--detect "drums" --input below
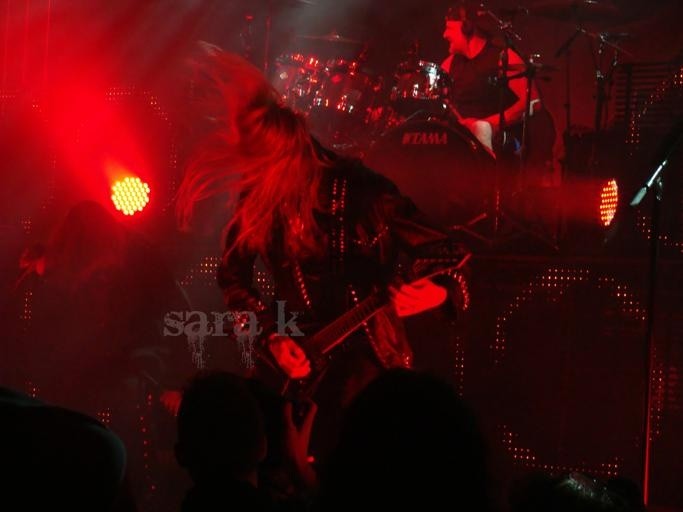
[304,59,384,152]
[391,60,454,116]
[369,123,478,227]
[272,51,324,109]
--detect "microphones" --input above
[627,152,671,207]
[553,31,579,58]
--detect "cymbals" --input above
[304,35,360,48]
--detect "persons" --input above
[170,369,304,510]
[424,3,543,178]
[0,385,128,510]
[293,365,503,511]
[174,47,485,471]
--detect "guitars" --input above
[159,254,471,488]
